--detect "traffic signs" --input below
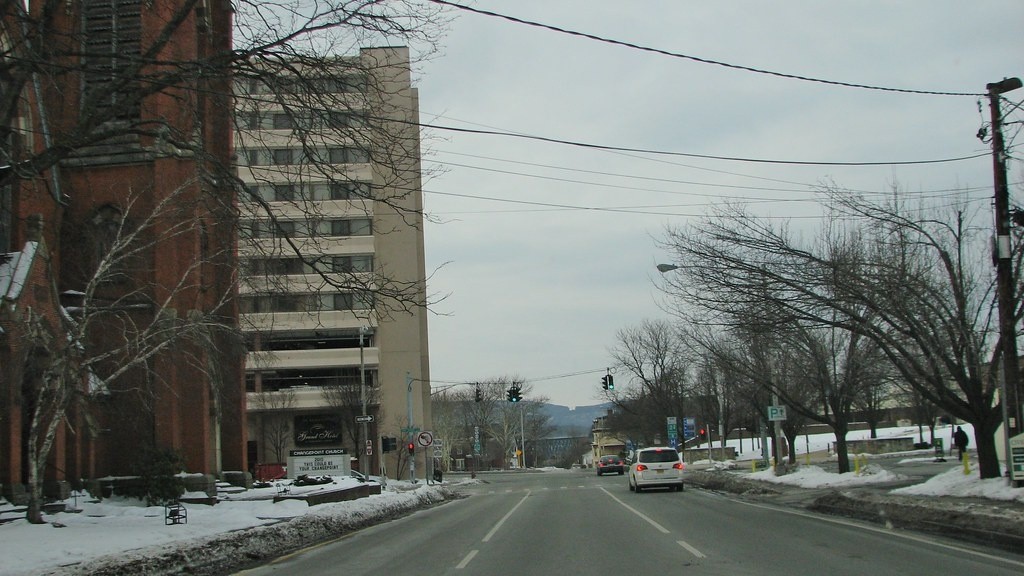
[356,415,374,423]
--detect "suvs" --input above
[629,446,685,491]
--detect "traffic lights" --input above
[609,376,614,389]
[507,388,523,403]
[382,437,397,453]
[701,428,706,441]
[476,388,481,402]
[602,377,608,390]
[409,443,415,456]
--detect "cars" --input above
[351,470,386,489]
[596,455,625,476]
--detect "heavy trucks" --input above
[255,458,357,481]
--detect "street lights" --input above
[520,401,525,467]
[658,263,790,479]
[359,324,371,482]
[600,406,604,454]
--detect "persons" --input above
[955,426,968,460]
